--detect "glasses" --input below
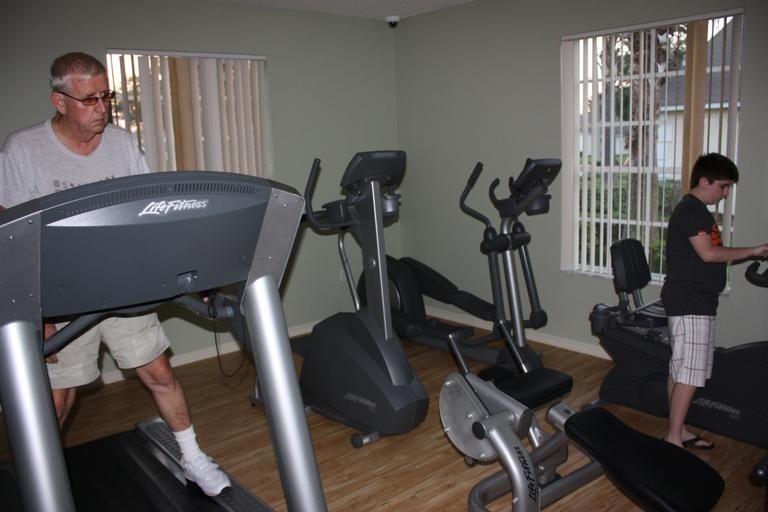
[58,90,115,107]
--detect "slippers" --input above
[682,434,715,450]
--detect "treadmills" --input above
[0,167,325,512]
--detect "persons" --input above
[658,152,768,449]
[0,51,233,499]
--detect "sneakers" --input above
[180,451,232,498]
